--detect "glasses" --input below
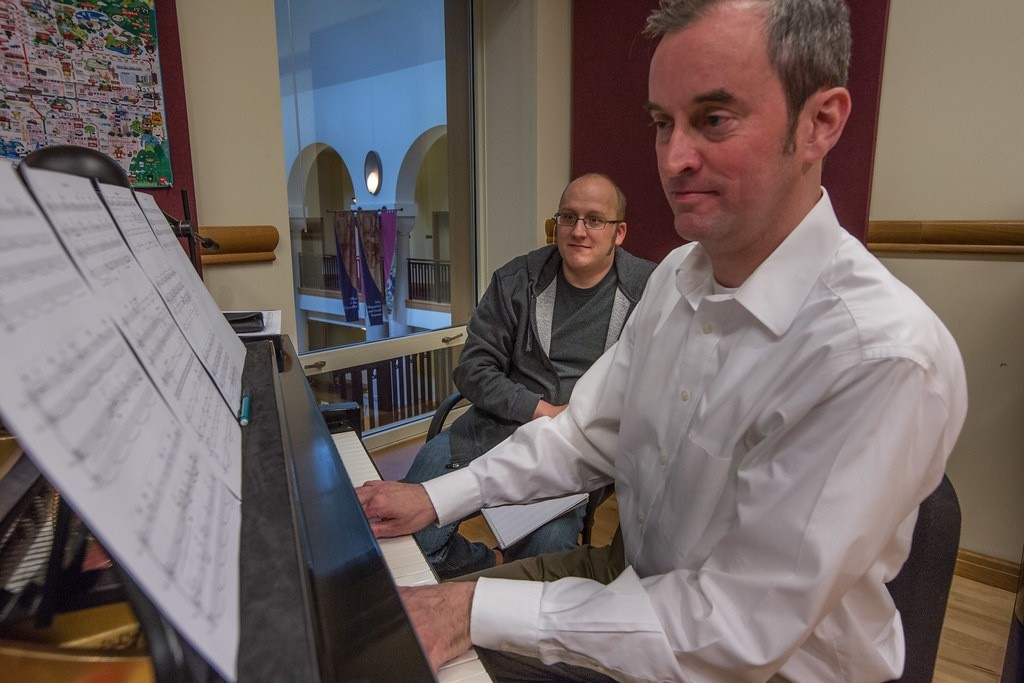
[553,212,623,230]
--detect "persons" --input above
[393,173,660,585]
[354,0,970,683]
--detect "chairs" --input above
[863,469,962,682]
[425,395,619,546]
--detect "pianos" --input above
[1,144,499,682]
